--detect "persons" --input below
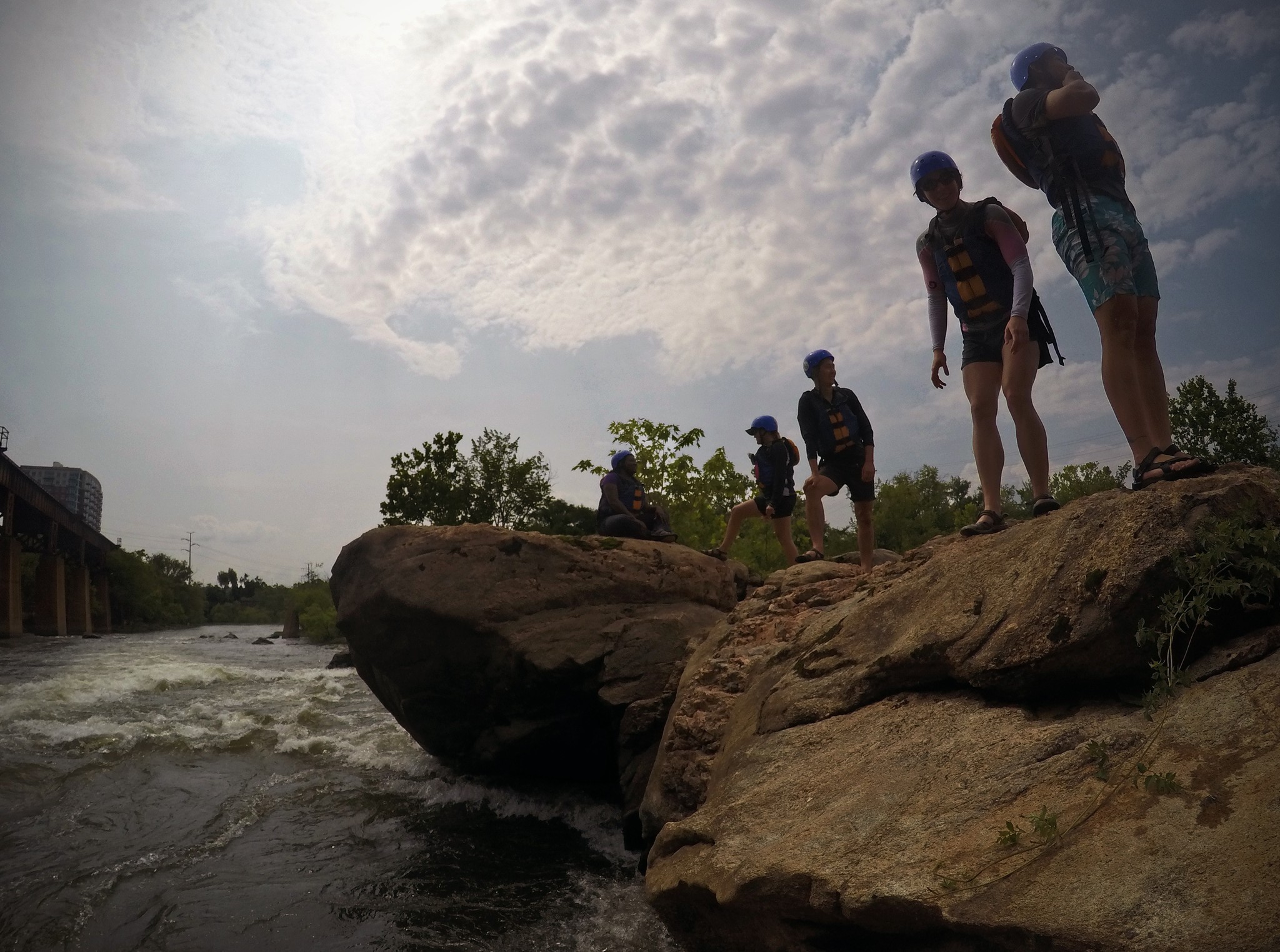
[597,450,679,543]
[700,415,799,569]
[795,350,876,573]
[1001,42,1209,493]
[910,151,1066,538]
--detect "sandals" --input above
[795,548,825,563]
[1034,495,1061,517]
[650,528,677,543]
[960,510,1008,536]
[1132,447,1202,491]
[1164,445,1216,475]
[700,548,727,561]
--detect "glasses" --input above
[919,172,956,191]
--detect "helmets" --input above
[911,151,963,202]
[611,450,635,470]
[803,350,834,378]
[1010,42,1067,92]
[745,415,778,436]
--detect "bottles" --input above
[754,461,759,478]
[633,482,642,510]
